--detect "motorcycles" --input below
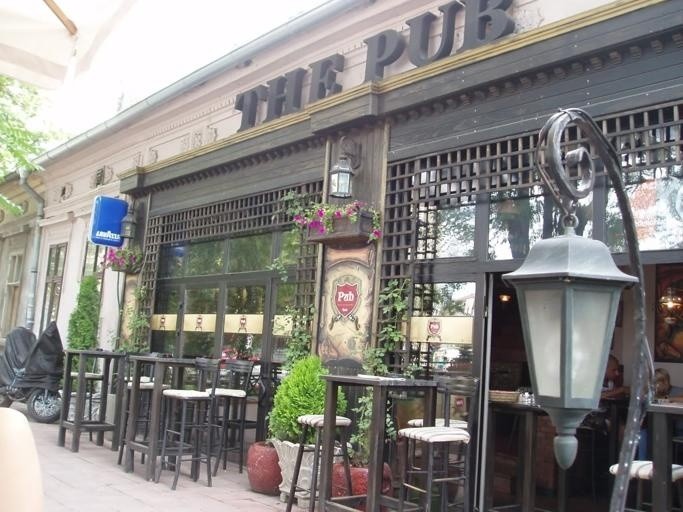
[1,320,63,424]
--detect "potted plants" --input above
[269,352,397,512]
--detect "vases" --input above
[248,439,282,494]
[111,264,131,272]
[305,212,377,243]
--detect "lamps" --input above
[120,204,139,239]
[329,133,363,199]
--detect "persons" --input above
[591,353,631,463]
[654,368,682,403]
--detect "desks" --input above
[318,369,436,511]
[59,349,124,452]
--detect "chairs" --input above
[71,349,281,491]
[400,373,681,511]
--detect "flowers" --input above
[266,190,382,282]
[100,244,142,275]
